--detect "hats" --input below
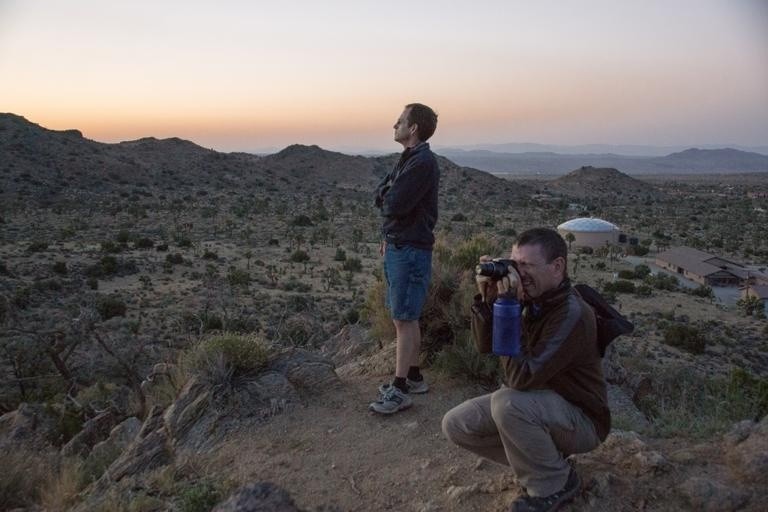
[573,284,635,359]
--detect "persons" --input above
[438,225,613,510]
[367,102,439,417]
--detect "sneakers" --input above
[507,467,582,512]
[369,384,414,415]
[378,376,430,396]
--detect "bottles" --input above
[492,298,520,356]
